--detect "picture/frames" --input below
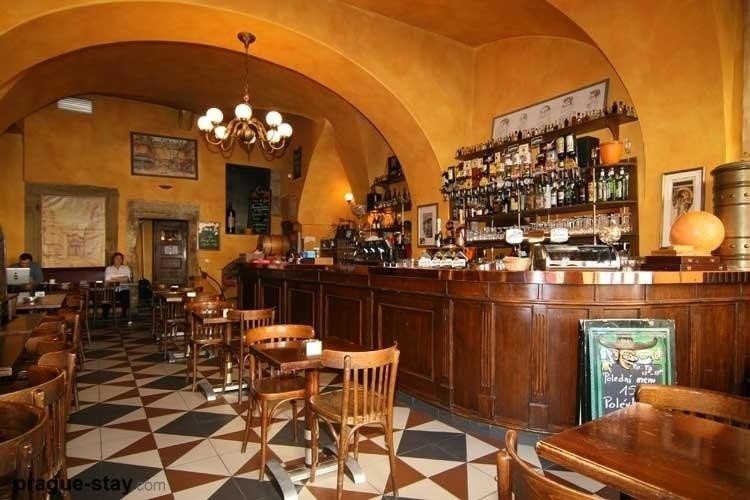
[659,166,706,249]
[130,130,199,179]
[491,78,610,144]
[197,220,220,250]
[416,203,438,248]
[576,319,677,427]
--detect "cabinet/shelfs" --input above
[442,113,640,270]
[364,174,412,236]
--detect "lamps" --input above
[345,193,353,205]
[196,33,294,155]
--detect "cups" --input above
[621,257,641,272]
[466,212,631,244]
[160,285,165,289]
[395,258,418,268]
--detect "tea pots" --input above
[330,239,337,265]
[320,239,329,248]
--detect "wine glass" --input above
[383,232,402,267]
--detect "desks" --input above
[535,401,750,500]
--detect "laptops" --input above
[5,268,31,284]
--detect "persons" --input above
[422,214,432,237]
[105,253,132,322]
[7,254,44,294]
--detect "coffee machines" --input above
[351,240,405,266]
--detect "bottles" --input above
[455,209,466,248]
[374,175,385,184]
[434,218,443,247]
[225,200,236,234]
[372,212,399,229]
[440,99,637,220]
[374,186,408,209]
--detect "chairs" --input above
[494,430,607,500]
[0,278,130,500]
[147,274,400,500]
[634,383,749,428]
